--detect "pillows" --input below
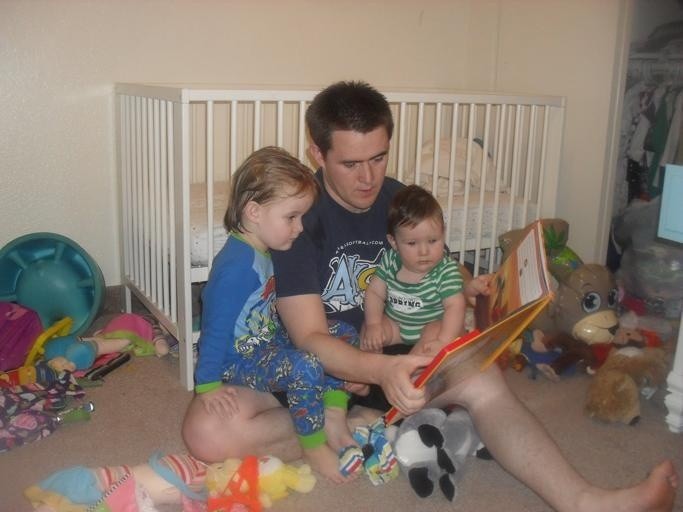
[412,134,478,184]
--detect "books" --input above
[382,217,554,425]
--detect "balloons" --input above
[497,217,617,346]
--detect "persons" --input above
[356,184,469,361]
[178,79,677,512]
[191,145,360,485]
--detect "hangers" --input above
[625,53,681,100]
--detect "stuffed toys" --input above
[23,451,207,512]
[383,405,493,503]
[202,455,313,512]
[504,319,668,432]
[43,311,178,373]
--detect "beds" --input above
[111,76,568,391]
